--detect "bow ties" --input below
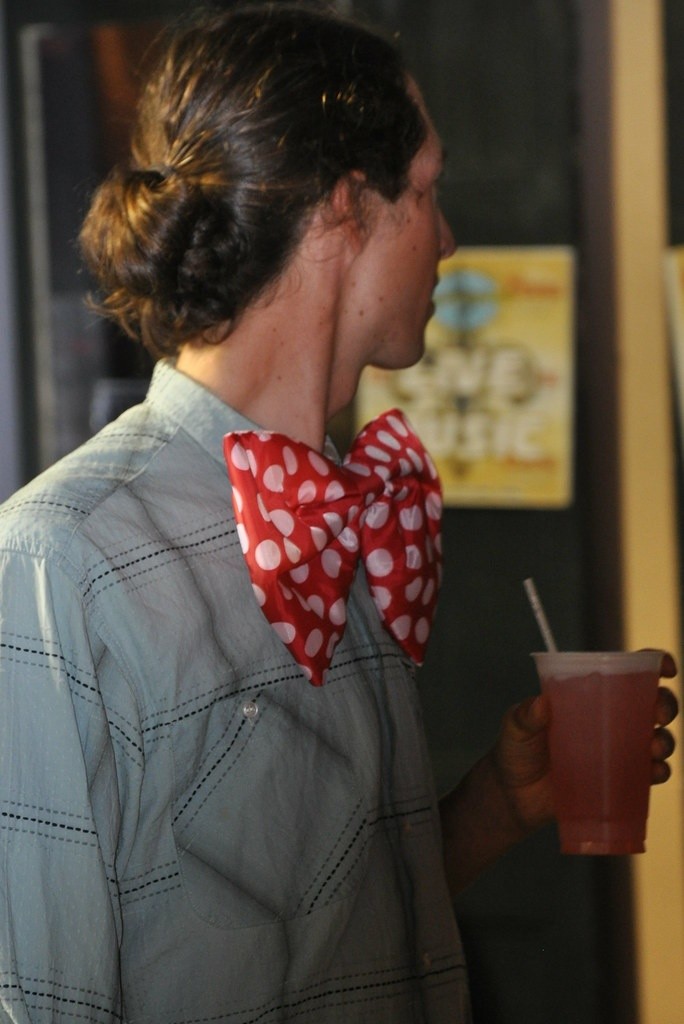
[225,407,448,690]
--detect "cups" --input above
[528,649,665,856]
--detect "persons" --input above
[0,1,679,1023]
